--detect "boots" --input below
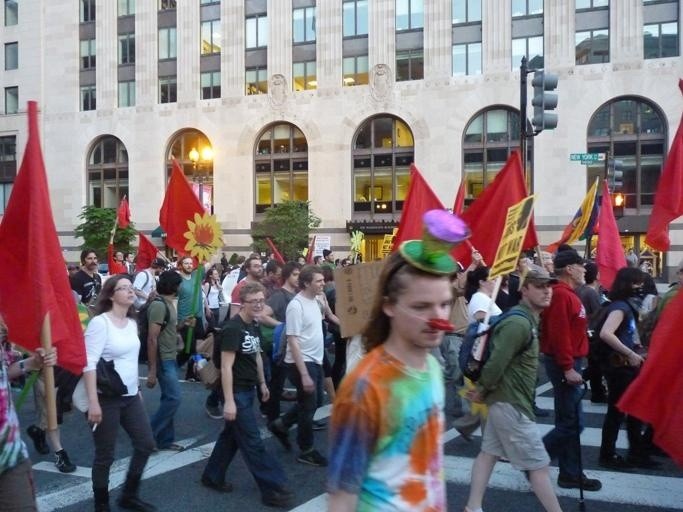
[117,472,156,512]
[93,482,113,512]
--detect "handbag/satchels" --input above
[259,313,276,355]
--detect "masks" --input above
[632,286,645,296]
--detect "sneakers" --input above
[590,392,608,403]
[598,452,638,472]
[556,471,601,491]
[452,419,475,441]
[626,450,664,471]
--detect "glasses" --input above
[244,299,265,305]
[115,287,134,290]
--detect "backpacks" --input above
[189,320,244,389]
[587,302,615,365]
[136,299,169,363]
[458,311,533,382]
[272,298,303,364]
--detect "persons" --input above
[0,228,682,512]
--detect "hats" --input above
[153,258,170,269]
[553,250,589,266]
[323,249,332,257]
[525,270,559,287]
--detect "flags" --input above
[543,176,600,254]
[158,159,212,273]
[595,178,628,294]
[389,163,474,275]
[0,100,87,376]
[456,148,539,269]
[117,198,131,232]
[450,180,464,217]
[641,110,683,254]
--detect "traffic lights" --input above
[535,69,562,130]
[610,158,625,188]
[614,192,625,216]
